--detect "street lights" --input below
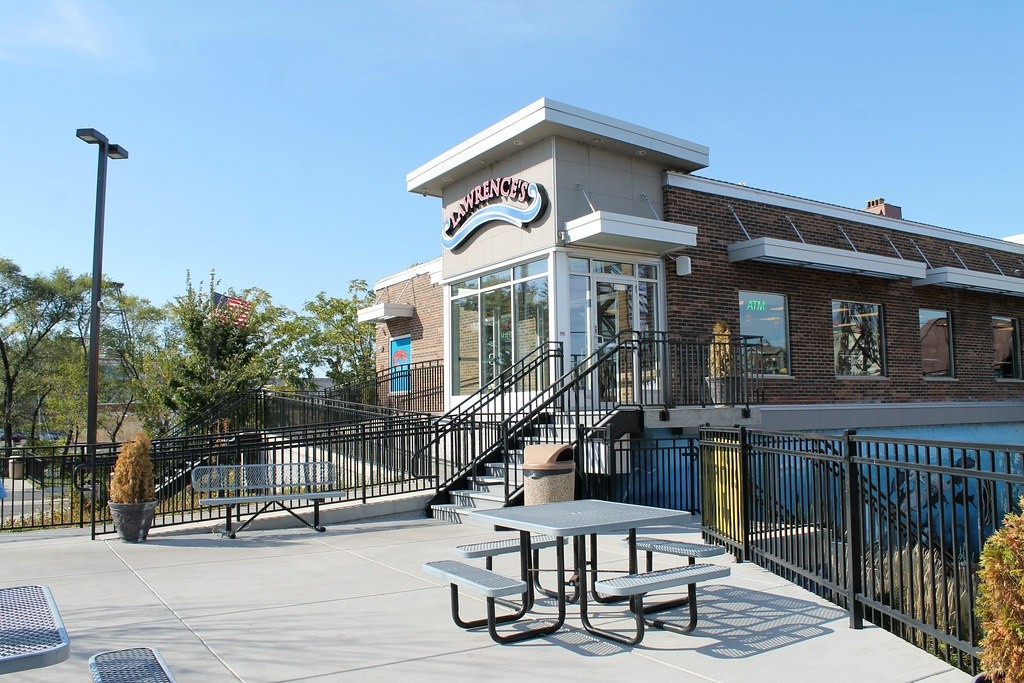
[75,126,130,485]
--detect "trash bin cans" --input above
[9,455,23,479]
[522,444,576,505]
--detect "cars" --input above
[0,428,67,445]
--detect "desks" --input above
[0,585,70,675]
[469,499,692,628]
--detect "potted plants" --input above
[706,321,743,407]
[108,431,158,544]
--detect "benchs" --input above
[88,647,176,683]
[192,462,346,538]
[425,535,732,647]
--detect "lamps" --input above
[423,189,429,197]
[558,230,565,241]
